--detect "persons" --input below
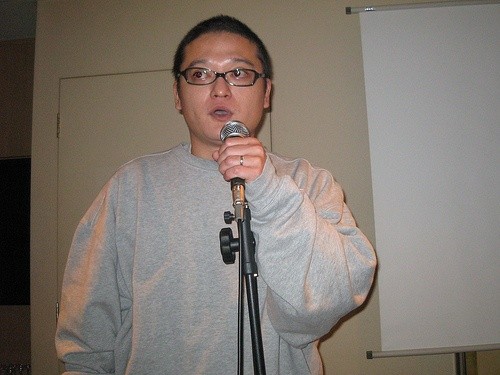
[53,13,378,374]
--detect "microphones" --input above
[220,119,251,221]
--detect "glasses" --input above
[177,67,267,87]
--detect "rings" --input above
[240,156,244,166]
